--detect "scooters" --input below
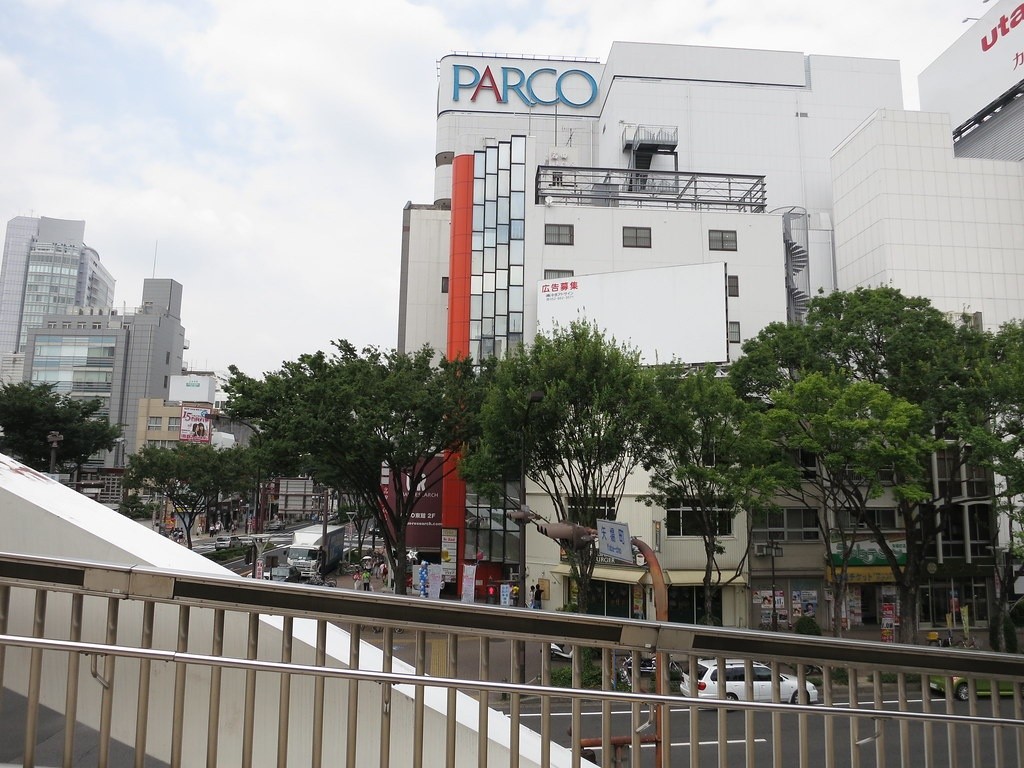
[551,642,573,661]
[622,652,683,680]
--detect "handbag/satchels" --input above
[509,589,519,598]
[356,579,360,587]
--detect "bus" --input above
[361,555,372,571]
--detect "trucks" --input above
[283,524,345,579]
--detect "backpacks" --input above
[383,565,388,574]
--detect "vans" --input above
[214,536,243,551]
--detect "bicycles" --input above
[927,630,954,647]
[303,573,338,587]
[954,634,979,650]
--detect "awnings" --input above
[662,571,750,588]
[548,562,647,592]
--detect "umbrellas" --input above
[362,556,372,559]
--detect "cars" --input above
[680,658,818,710]
[267,520,285,531]
[926,674,1024,700]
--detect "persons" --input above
[529,584,544,609]
[192,423,208,436]
[353,552,387,591]
[197,521,220,537]
[753,591,816,618]
[169,527,183,542]
[228,520,238,536]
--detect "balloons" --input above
[418,561,428,598]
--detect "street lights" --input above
[204,413,262,579]
[763,538,784,635]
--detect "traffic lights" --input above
[504,511,529,522]
[486,583,497,605]
[536,521,587,543]
